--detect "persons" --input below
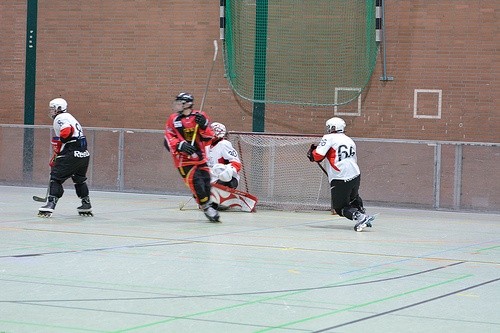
[163,92,222,223]
[36,97,94,218]
[203,120,242,190]
[306,117,375,232]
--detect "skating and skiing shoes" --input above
[38,196,58,218]
[200,203,220,222]
[77,197,93,216]
[353,212,375,232]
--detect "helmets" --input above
[211,122,226,138]
[326,117,346,133]
[176,93,194,102]
[49,98,67,112]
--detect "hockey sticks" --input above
[33,158,57,202]
[179,178,218,211]
[317,161,371,226]
[187,40,218,159]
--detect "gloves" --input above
[195,114,207,130]
[177,141,196,155]
[307,144,316,162]
[52,140,57,152]
